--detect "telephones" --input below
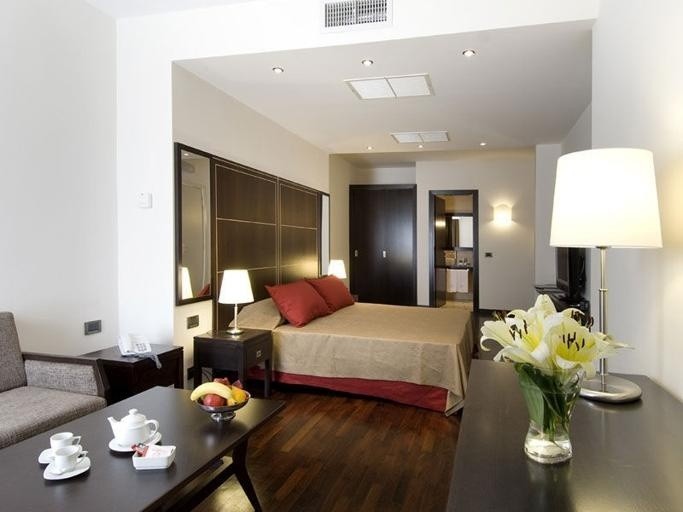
[118,332,152,357]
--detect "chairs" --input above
[0,310,107,448]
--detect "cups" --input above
[49,430,81,450]
[53,447,86,468]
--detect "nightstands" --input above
[351,293,359,303]
[192,324,275,400]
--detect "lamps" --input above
[181,266,194,302]
[550,146,661,408]
[488,201,516,230]
[217,268,254,334]
[327,258,347,281]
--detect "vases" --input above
[517,362,580,461]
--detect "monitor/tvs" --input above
[555,247,585,299]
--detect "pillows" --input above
[308,273,354,311]
[263,277,333,328]
[197,284,210,297]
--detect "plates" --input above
[43,458,90,481]
[106,433,163,454]
[36,445,83,464]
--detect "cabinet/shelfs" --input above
[348,184,417,307]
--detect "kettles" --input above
[106,407,159,445]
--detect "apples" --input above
[230,380,242,391]
[203,394,225,406]
[214,378,229,386]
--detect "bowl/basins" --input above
[131,445,177,470]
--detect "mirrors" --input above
[173,140,214,307]
[447,214,473,250]
[320,191,330,278]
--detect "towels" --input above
[442,269,469,295]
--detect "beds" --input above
[226,286,473,416]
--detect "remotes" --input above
[543,289,561,292]
[535,285,557,289]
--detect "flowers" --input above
[474,292,631,370]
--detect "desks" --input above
[445,357,681,512]
[80,341,183,404]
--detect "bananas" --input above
[231,386,245,405]
[190,382,236,406]
